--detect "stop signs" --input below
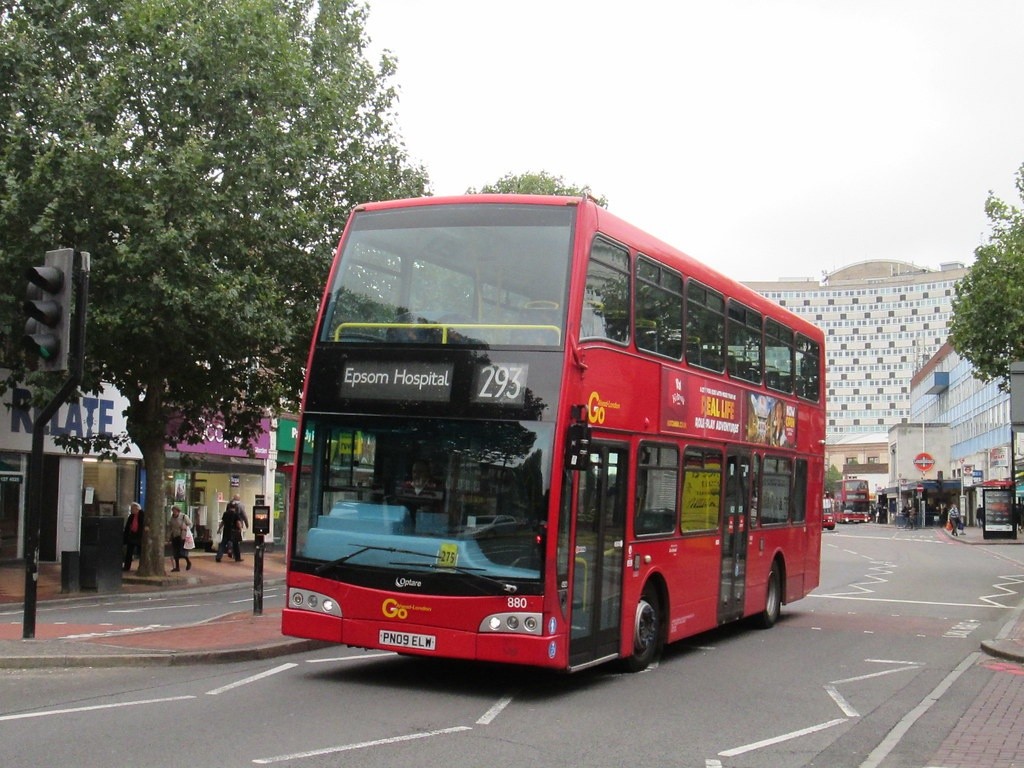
[916,483,924,493]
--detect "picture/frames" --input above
[96,501,116,516]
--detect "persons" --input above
[167,503,197,573]
[122,499,144,573]
[214,493,248,562]
[899,506,920,529]
[1016,498,1024,533]
[976,504,983,527]
[771,398,788,448]
[399,458,442,491]
[933,503,962,537]
[870,503,888,524]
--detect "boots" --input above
[184,557,192,570]
[171,562,180,571]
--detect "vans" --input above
[822,495,836,531]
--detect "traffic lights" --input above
[24,248,75,374]
[22,262,45,369]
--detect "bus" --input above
[279,192,827,675]
[832,478,871,524]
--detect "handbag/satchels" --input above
[946,522,952,531]
[231,530,242,542]
[183,525,195,549]
[958,522,963,530]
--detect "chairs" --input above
[468,308,522,346]
[382,309,478,345]
[514,299,806,399]
[643,507,675,530]
[726,475,748,499]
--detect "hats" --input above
[171,504,182,511]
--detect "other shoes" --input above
[122,566,131,571]
[217,559,221,562]
[235,558,243,561]
